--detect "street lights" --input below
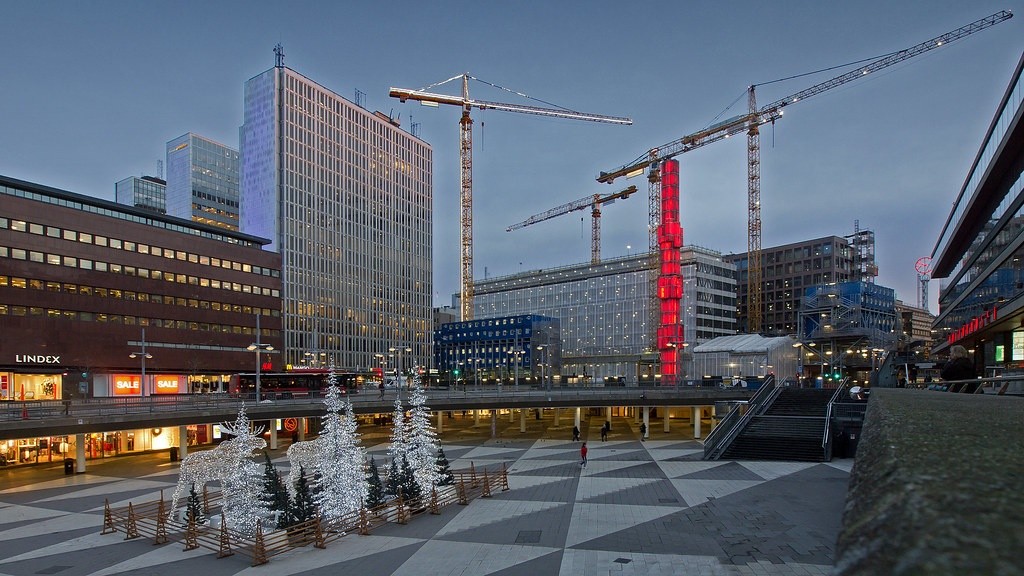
[129,352,153,400]
[508,349,526,386]
[537,344,551,391]
[247,343,274,404]
[389,346,412,400]
[375,354,385,385]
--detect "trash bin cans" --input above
[281,392,292,400]
[292,431,299,443]
[170,447,177,462]
[65,458,74,475]
[536,412,539,419]
[618,377,626,386]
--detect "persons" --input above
[292,429,300,443]
[940,345,984,394]
[601,426,608,442]
[60,394,73,415]
[910,367,918,383]
[579,443,587,468]
[377,382,385,400]
[572,426,580,441]
[639,423,646,441]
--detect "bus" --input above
[228,369,347,399]
[288,370,359,394]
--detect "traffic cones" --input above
[22,404,29,419]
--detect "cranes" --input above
[596,106,785,350]
[681,9,1014,331]
[389,71,633,319]
[508,185,639,264]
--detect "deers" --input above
[168,417,267,528]
